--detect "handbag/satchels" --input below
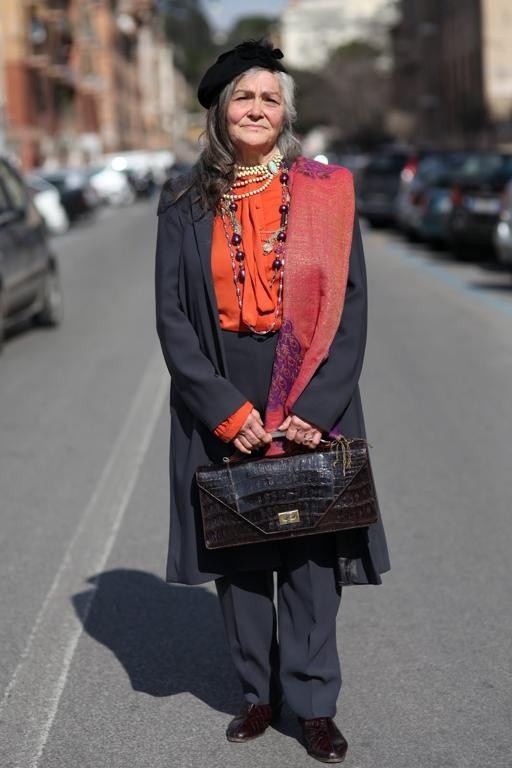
[189,429,380,552]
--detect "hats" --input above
[193,40,287,114]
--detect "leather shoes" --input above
[291,707,352,763]
[223,688,280,749]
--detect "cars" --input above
[311,139,512,268]
[0,146,178,337]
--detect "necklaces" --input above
[212,148,291,340]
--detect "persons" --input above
[153,34,393,764]
